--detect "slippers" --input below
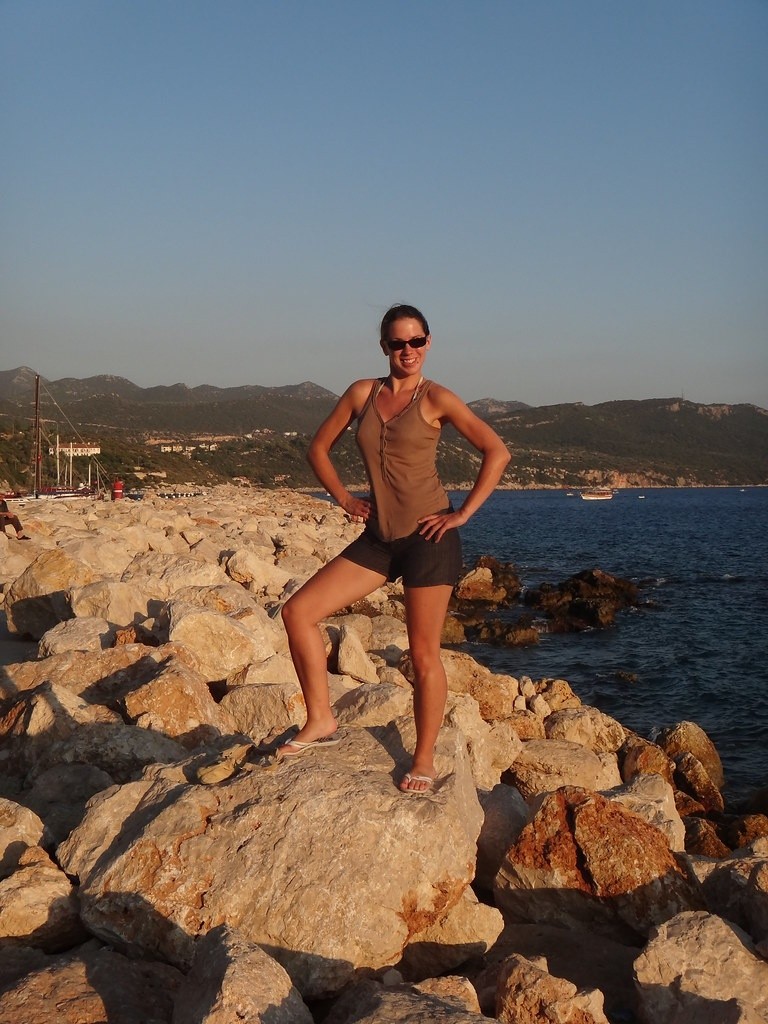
[399,772,435,794]
[279,733,341,756]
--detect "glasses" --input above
[384,334,427,351]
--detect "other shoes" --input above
[18,535,31,540]
[6,535,12,539]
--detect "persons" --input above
[273,304,513,793]
[0,489,31,540]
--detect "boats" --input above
[580,489,613,500]
